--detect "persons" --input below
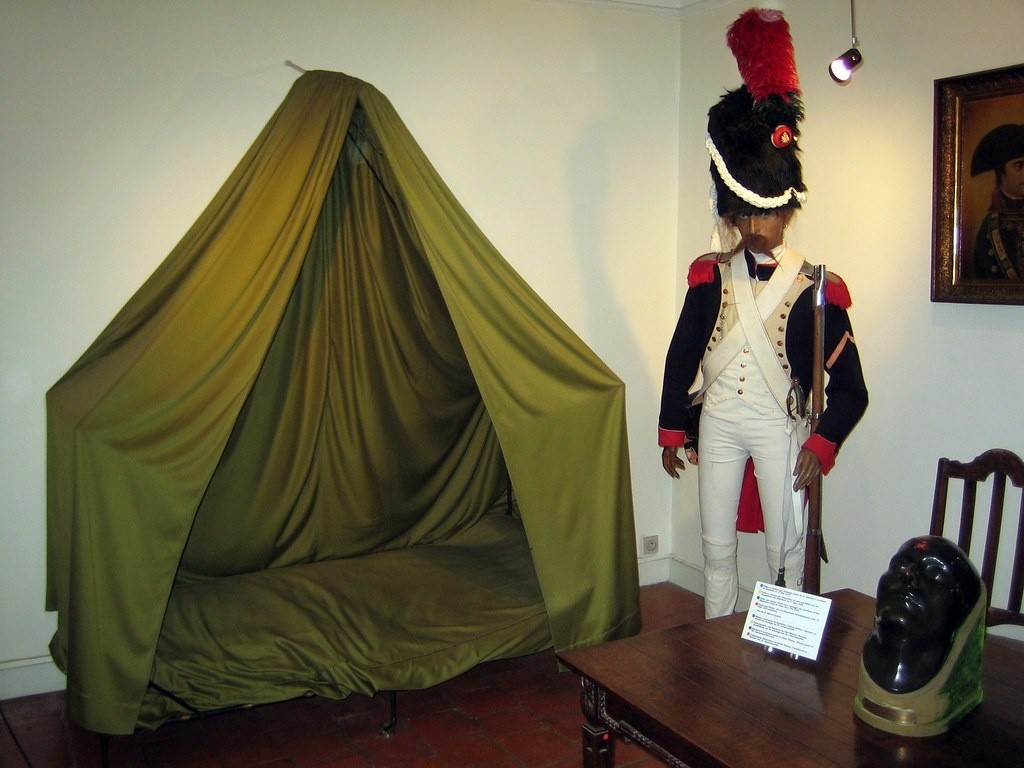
[656,7,869,620]
[855,534,988,738]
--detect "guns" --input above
[800,262,829,600]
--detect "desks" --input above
[556,589,1024,768]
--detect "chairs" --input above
[930,449,1024,629]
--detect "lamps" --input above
[828,0,862,82]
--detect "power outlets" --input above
[643,536,659,555]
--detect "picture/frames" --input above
[931,63,1024,306]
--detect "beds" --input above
[46,384,642,739]
